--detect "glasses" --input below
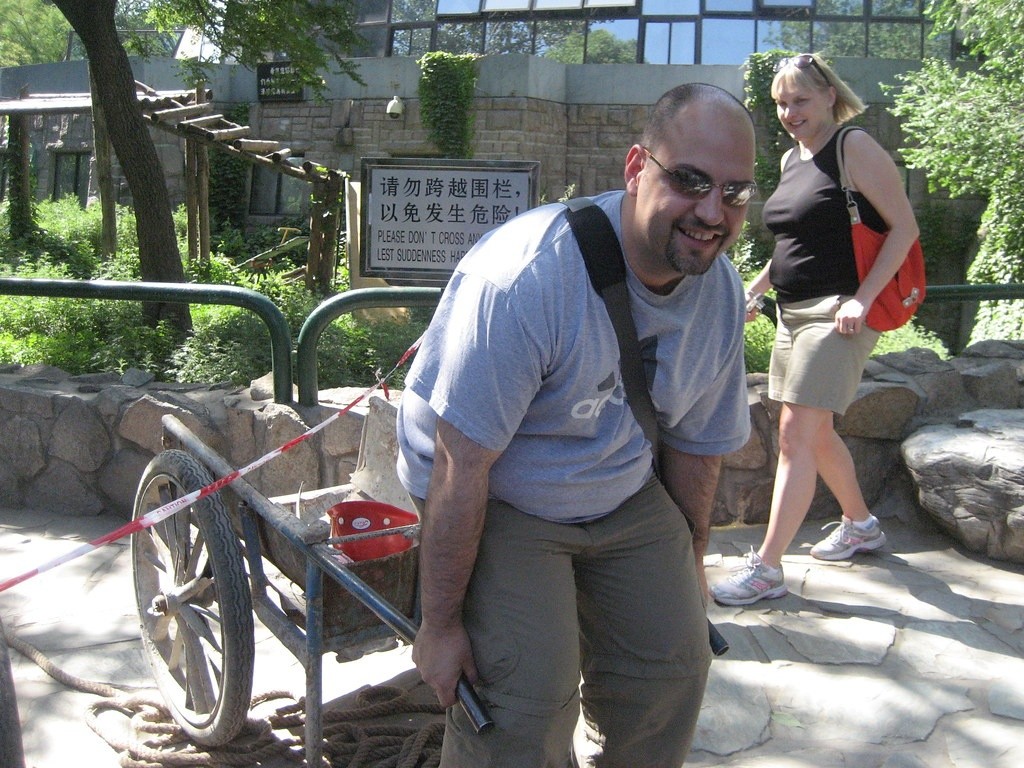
[772,55,831,88]
[641,146,758,206]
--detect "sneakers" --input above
[709,544,787,605]
[810,514,885,561]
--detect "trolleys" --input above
[128,412,731,768]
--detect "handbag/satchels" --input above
[836,125,926,331]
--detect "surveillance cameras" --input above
[386,100,402,119]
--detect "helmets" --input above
[327,501,420,561]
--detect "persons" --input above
[395,82,756,768]
[709,54,919,606]
